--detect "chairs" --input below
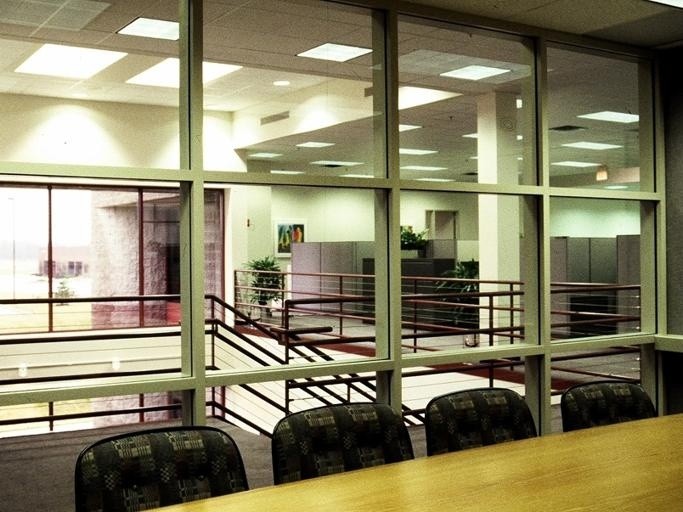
[75,425,249,511]
[560,382,657,433]
[271,401,415,486]
[423,387,538,457]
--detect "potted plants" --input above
[435,258,480,346]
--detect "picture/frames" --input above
[275,220,309,258]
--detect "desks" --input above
[140,411,683,512]
